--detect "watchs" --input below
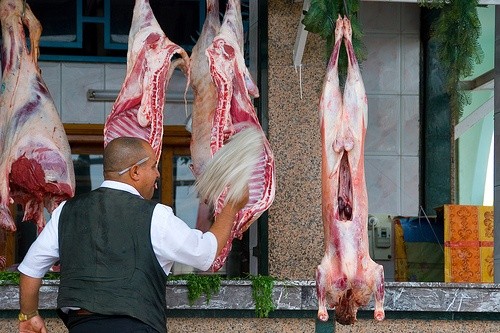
[19,310,38,321]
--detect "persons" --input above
[17,137,249,333]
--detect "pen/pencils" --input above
[119,156,150,176]
